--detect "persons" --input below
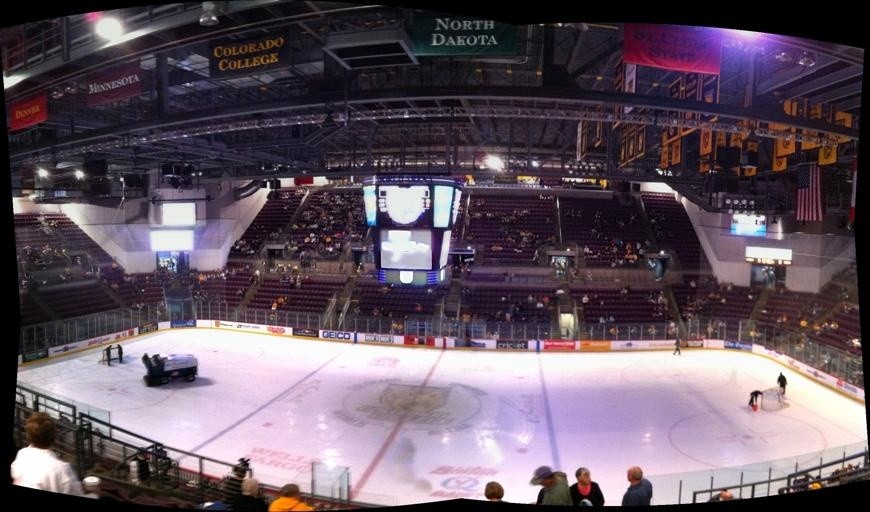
[777,372,788,395]
[672,334,681,355]
[568,466,605,507]
[789,452,869,493]
[115,343,123,364]
[14,209,179,350]
[621,465,653,507]
[530,465,574,505]
[484,480,504,502]
[708,489,734,503]
[536,471,569,504]
[105,344,115,366]
[748,390,763,407]
[736,242,865,389]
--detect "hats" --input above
[530,465,552,485]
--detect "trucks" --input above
[143,352,198,385]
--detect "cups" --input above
[83,475,101,492]
